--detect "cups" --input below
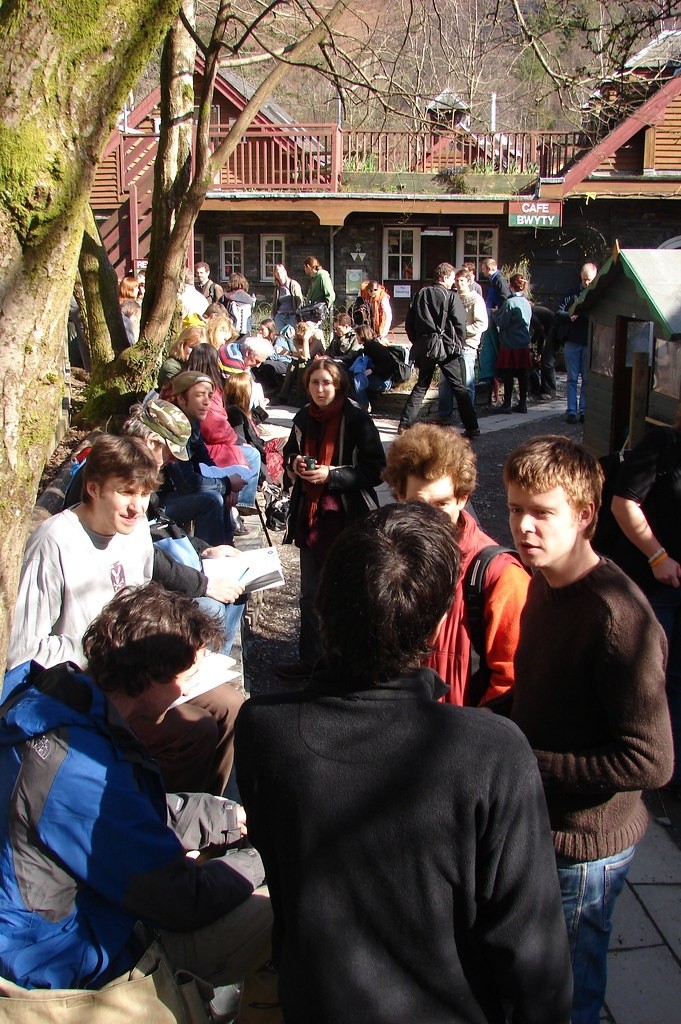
[303,455,316,470]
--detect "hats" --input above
[138,399,190,461]
[171,371,214,396]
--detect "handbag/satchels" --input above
[266,485,291,531]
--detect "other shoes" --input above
[580,415,584,423]
[512,406,527,413]
[460,430,480,438]
[233,514,251,535]
[568,414,576,423]
[496,407,512,413]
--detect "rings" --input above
[313,480,316,485]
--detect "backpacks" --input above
[379,346,411,389]
[593,450,632,553]
[409,333,447,363]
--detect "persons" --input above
[283,357,391,695]
[380,424,534,713]
[1,580,266,1024]
[554,261,601,424]
[586,426,681,828]
[483,434,673,1024]
[5,434,252,798]
[231,501,575,1024]
[64,240,564,574]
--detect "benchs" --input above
[275,168,331,176]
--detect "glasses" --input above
[370,289,377,291]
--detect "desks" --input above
[282,150,372,182]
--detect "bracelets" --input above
[648,547,668,568]
